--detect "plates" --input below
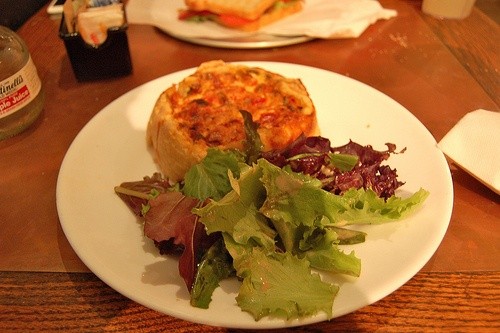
[57,60,453,330]
[437,105,499,199]
[153,1,320,48]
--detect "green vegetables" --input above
[114,111,428,323]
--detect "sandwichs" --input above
[177,0,304,32]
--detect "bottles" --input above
[0,24,42,140]
